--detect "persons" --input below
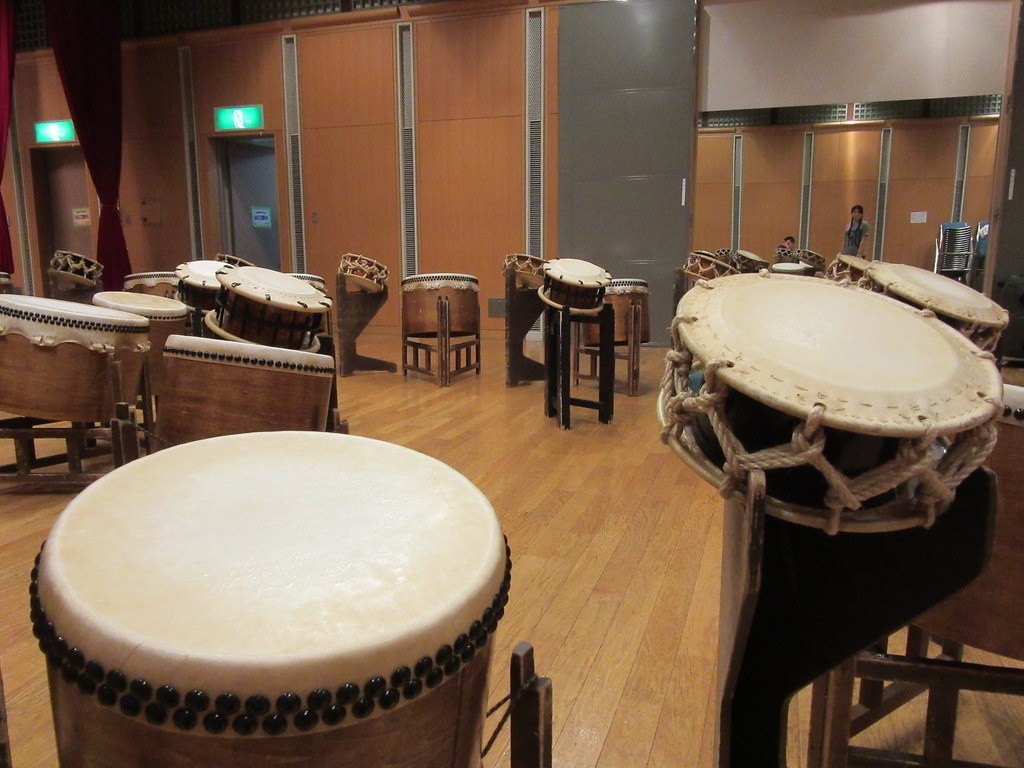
[842,205,870,259]
[784,236,795,263]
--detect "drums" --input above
[908,383,1024,662]
[124,252,332,353]
[836,254,871,288]
[337,253,388,287]
[537,258,612,315]
[772,263,805,275]
[776,248,795,258]
[92,291,187,396]
[582,278,650,346]
[683,248,737,280]
[502,253,546,287]
[401,272,480,338]
[0,293,150,422]
[148,334,335,453]
[795,249,826,268]
[733,250,769,273]
[48,250,103,285]
[655,273,1004,534]
[861,260,1009,355]
[28,429,514,768]
